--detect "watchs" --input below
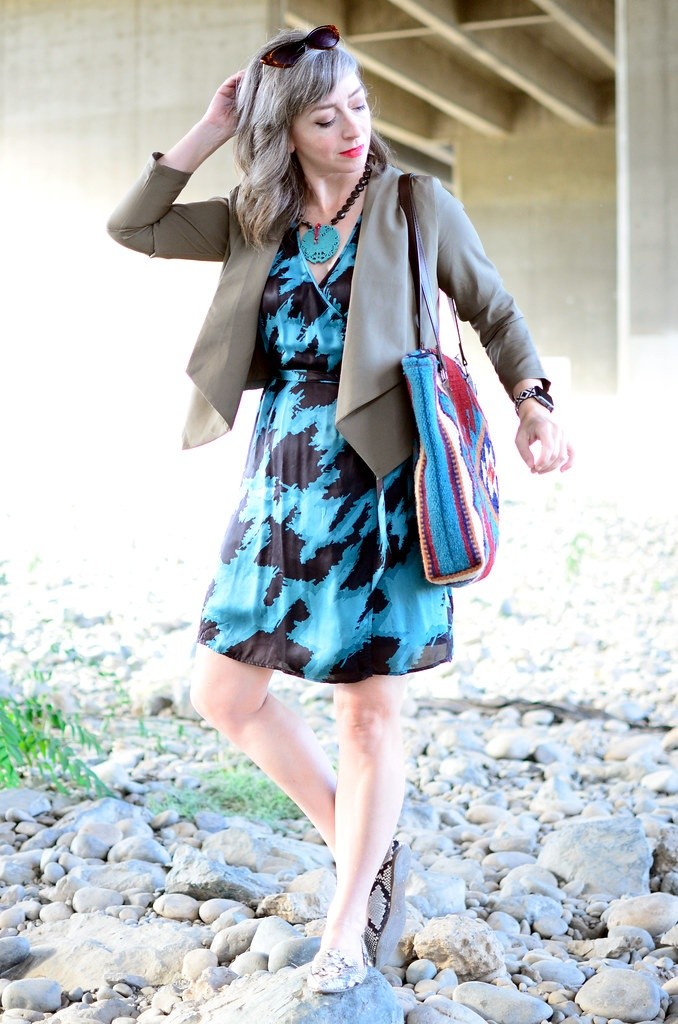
[515,386,554,416]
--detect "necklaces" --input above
[296,162,372,264]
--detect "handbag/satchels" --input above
[398,174,500,589]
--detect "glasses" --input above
[259,25,340,69]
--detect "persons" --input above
[108,32,574,995]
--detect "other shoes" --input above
[306,935,369,992]
[361,838,413,970]
